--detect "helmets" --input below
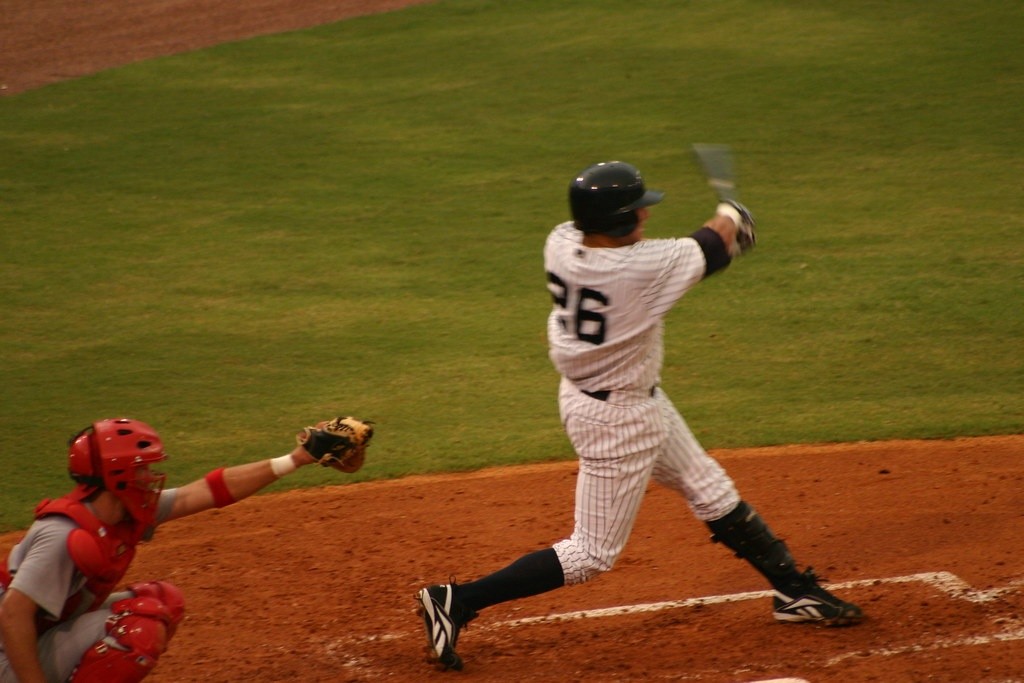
[568,161,663,237]
[68,419,170,525]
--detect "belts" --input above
[581,386,656,406]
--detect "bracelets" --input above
[271,453,295,476]
[204,466,236,509]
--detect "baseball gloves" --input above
[295,415,374,475]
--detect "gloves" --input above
[715,197,757,257]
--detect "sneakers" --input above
[413,575,479,672]
[773,566,863,625]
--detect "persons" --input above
[418,160,864,669]
[0,418,373,683]
